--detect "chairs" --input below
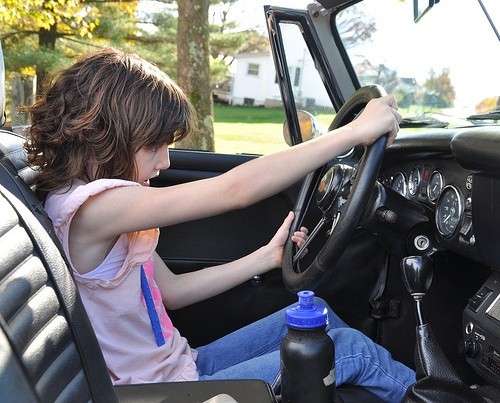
[0,148,120,403]
[0,40,76,286]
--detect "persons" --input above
[22,47,417,403]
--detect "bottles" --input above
[281,290,335,403]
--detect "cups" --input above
[11,74,36,137]
